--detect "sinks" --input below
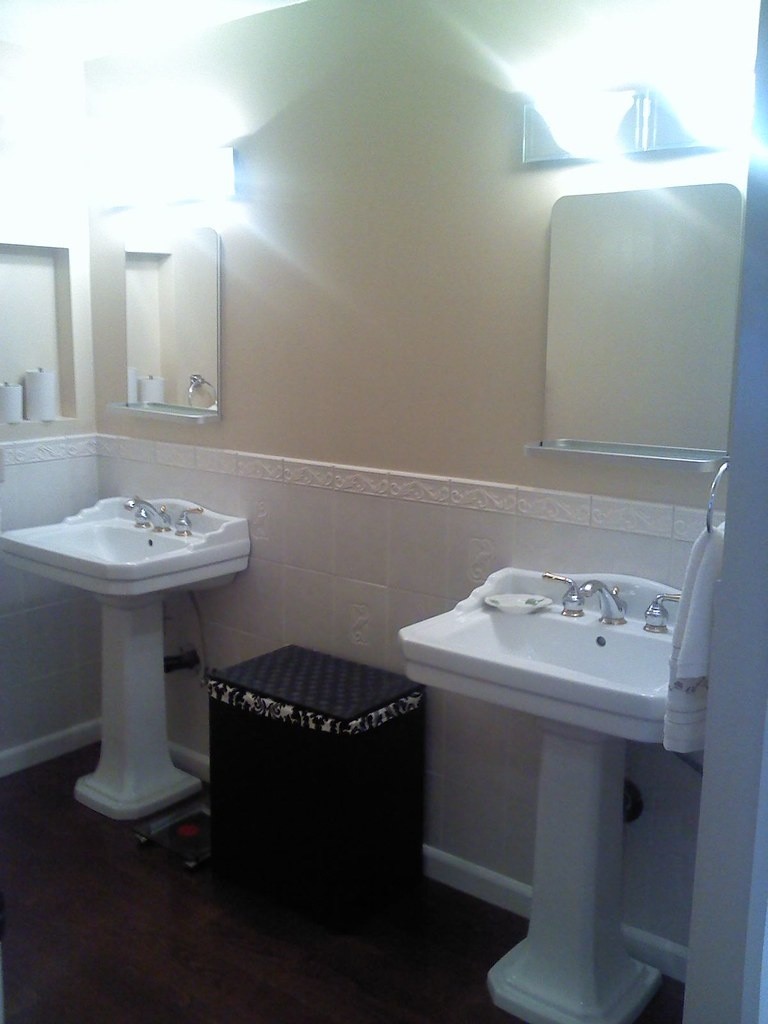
[0,495,251,609]
[396,566,683,746]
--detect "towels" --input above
[659,521,725,755]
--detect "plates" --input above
[486,594,552,614]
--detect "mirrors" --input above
[109,228,222,420]
[522,181,743,475]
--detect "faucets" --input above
[121,498,174,534]
[579,580,629,627]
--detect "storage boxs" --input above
[205,645,422,933]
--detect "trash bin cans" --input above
[206,644,428,927]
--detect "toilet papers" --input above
[0,367,58,424]
[127,366,166,404]
